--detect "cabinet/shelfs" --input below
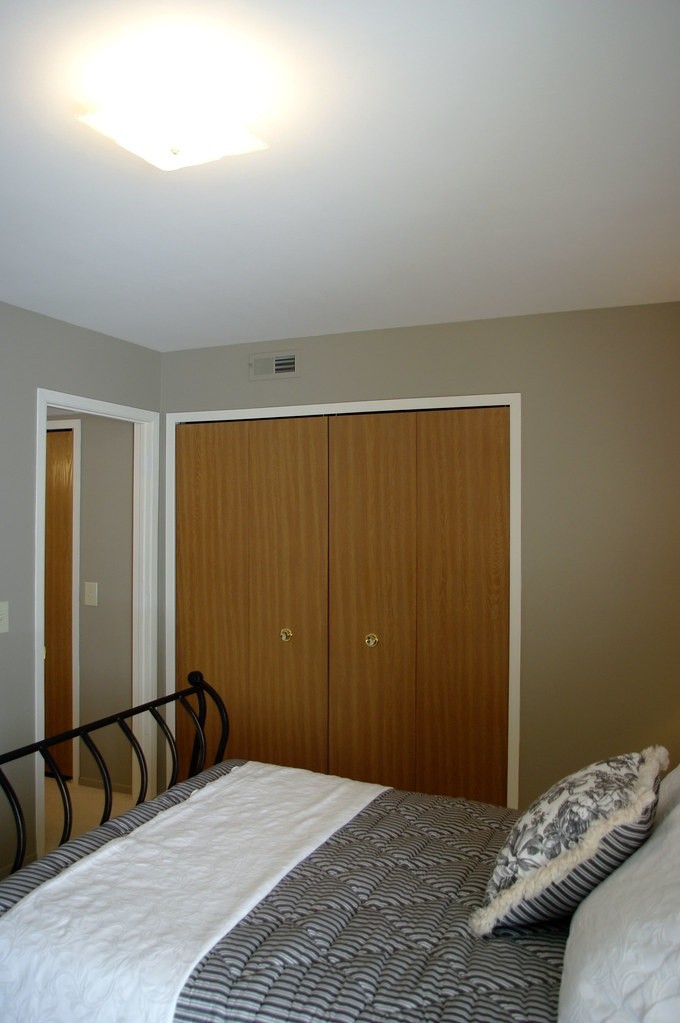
[172,407,510,808]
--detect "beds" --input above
[0,669,680,1023]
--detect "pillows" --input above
[469,744,670,941]
